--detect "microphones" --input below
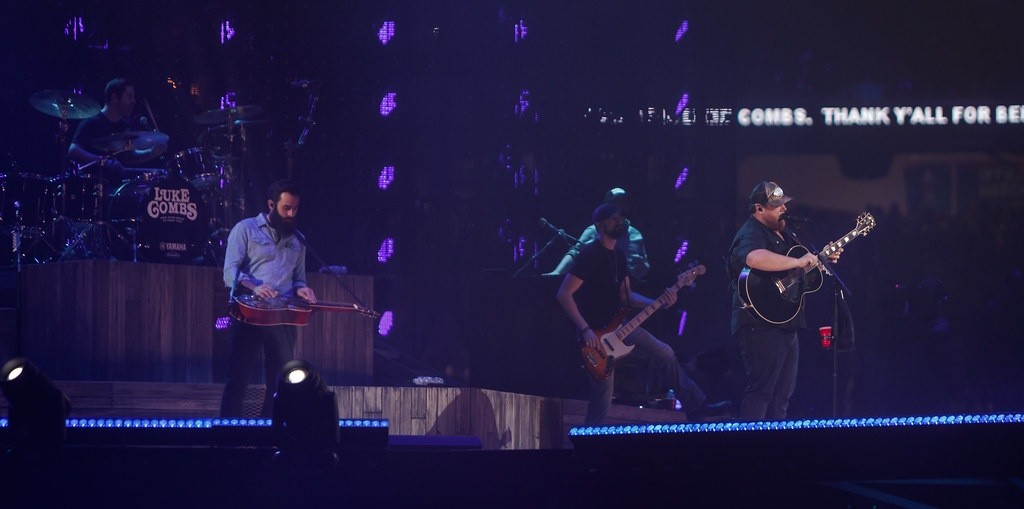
[539,217,567,241]
[546,228,564,246]
[785,214,813,224]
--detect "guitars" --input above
[736,210,878,326]
[575,259,707,381]
[227,291,383,328]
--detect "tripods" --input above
[209,113,234,257]
[11,138,133,268]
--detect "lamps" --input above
[269,359,337,483]
[0,357,72,493]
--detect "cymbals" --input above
[89,129,170,154]
[29,89,102,121]
[191,104,262,125]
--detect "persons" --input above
[540,186,732,430]
[68,77,168,176]
[220,181,319,417]
[726,179,846,419]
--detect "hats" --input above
[592,204,619,222]
[604,186,632,202]
[749,181,794,207]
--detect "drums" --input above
[51,172,102,221]
[171,145,221,189]
[103,172,211,266]
[0,172,61,228]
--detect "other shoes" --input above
[686,399,732,421]
